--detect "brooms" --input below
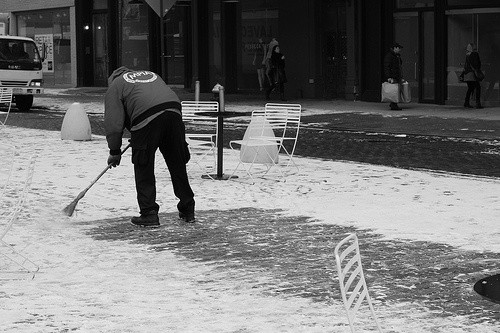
[63,142,131,216]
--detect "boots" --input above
[264,91,271,100]
[280,92,287,101]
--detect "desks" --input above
[195,112,252,180]
[474,273,500,304]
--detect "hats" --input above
[392,43,403,48]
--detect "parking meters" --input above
[212,83,225,176]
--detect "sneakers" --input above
[131,216,159,227]
[179,214,195,222]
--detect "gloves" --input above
[107,149,121,168]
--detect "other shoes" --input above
[391,107,401,110]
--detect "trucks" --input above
[0,35,46,113]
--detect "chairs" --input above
[335,234,382,333]
[0,87,39,279]
[181,101,301,186]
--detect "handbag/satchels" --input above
[381,80,401,103]
[474,70,484,81]
[400,81,411,103]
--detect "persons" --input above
[264,45,289,101]
[0,44,11,60]
[104,66,196,227]
[265,38,278,91]
[383,43,404,110]
[253,42,266,88]
[459,43,484,109]
